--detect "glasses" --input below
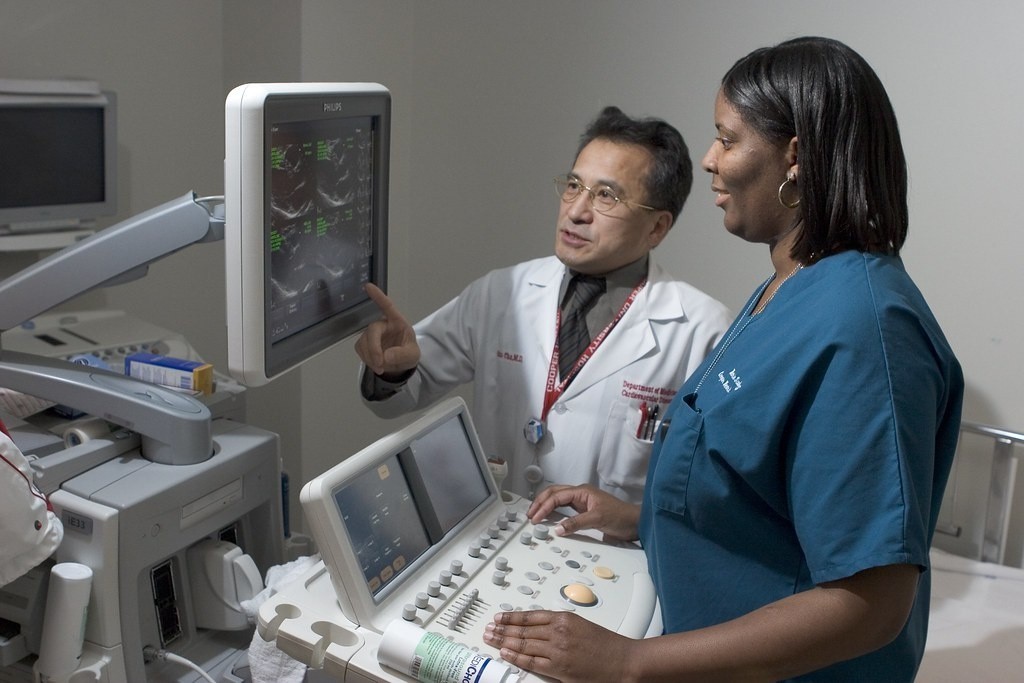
[553,173,659,212]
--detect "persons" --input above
[480,34,967,683]
[354,102,731,551]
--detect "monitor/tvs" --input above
[224,81,392,388]
[330,404,499,606]
[0,89,118,226]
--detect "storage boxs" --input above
[124,353,213,395]
